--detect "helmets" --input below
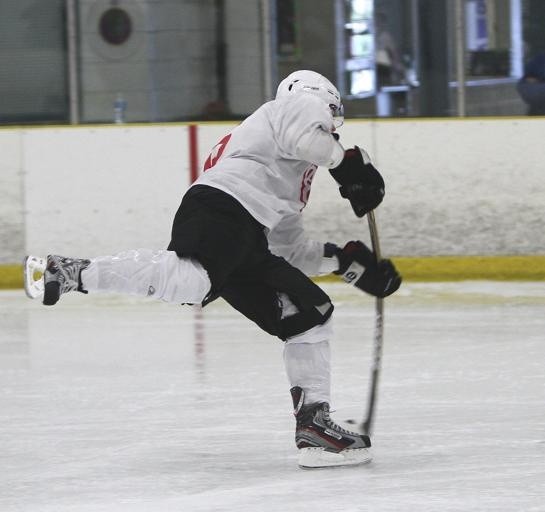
[276,69,340,109]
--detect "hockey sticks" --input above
[340,209,384,436]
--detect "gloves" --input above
[324,241,401,297]
[329,147,385,218]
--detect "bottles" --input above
[113,92,127,124]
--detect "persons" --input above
[375,12,403,85]
[44,70,401,452]
[516,26,545,116]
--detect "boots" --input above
[290,387,370,448]
[44,255,91,306]
